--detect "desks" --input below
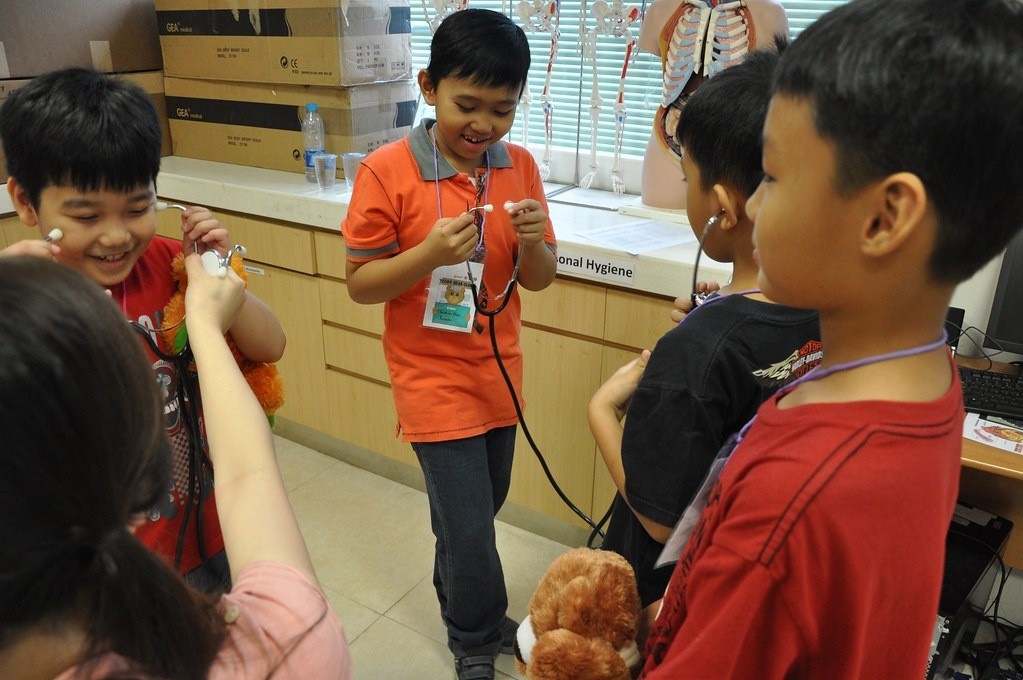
[952,351,1023,573]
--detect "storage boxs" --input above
[0,0,417,179]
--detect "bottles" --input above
[301,103,325,183]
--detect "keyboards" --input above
[954,366,1023,420]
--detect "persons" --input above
[1,238,352,679]
[635,1,1023,680]
[587,34,821,655]
[339,7,559,680]
[0,66,289,609]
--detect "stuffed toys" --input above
[512,546,645,680]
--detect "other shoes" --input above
[443,612,520,680]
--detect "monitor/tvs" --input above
[982,230,1023,355]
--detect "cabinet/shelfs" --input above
[0,197,680,551]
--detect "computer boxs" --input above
[927,499,1014,680]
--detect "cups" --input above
[341,152,367,190]
[312,154,338,193]
